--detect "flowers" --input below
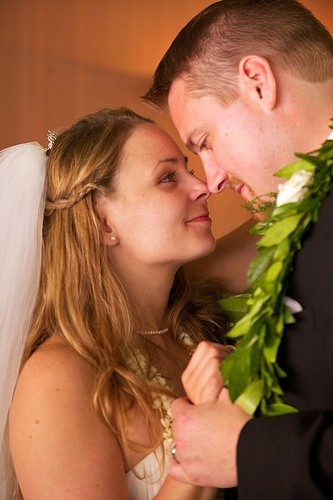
[221,114,333,414]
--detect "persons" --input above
[139,0,333,500]
[1,107,238,500]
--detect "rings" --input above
[171,446,180,463]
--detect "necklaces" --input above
[135,325,184,367]
